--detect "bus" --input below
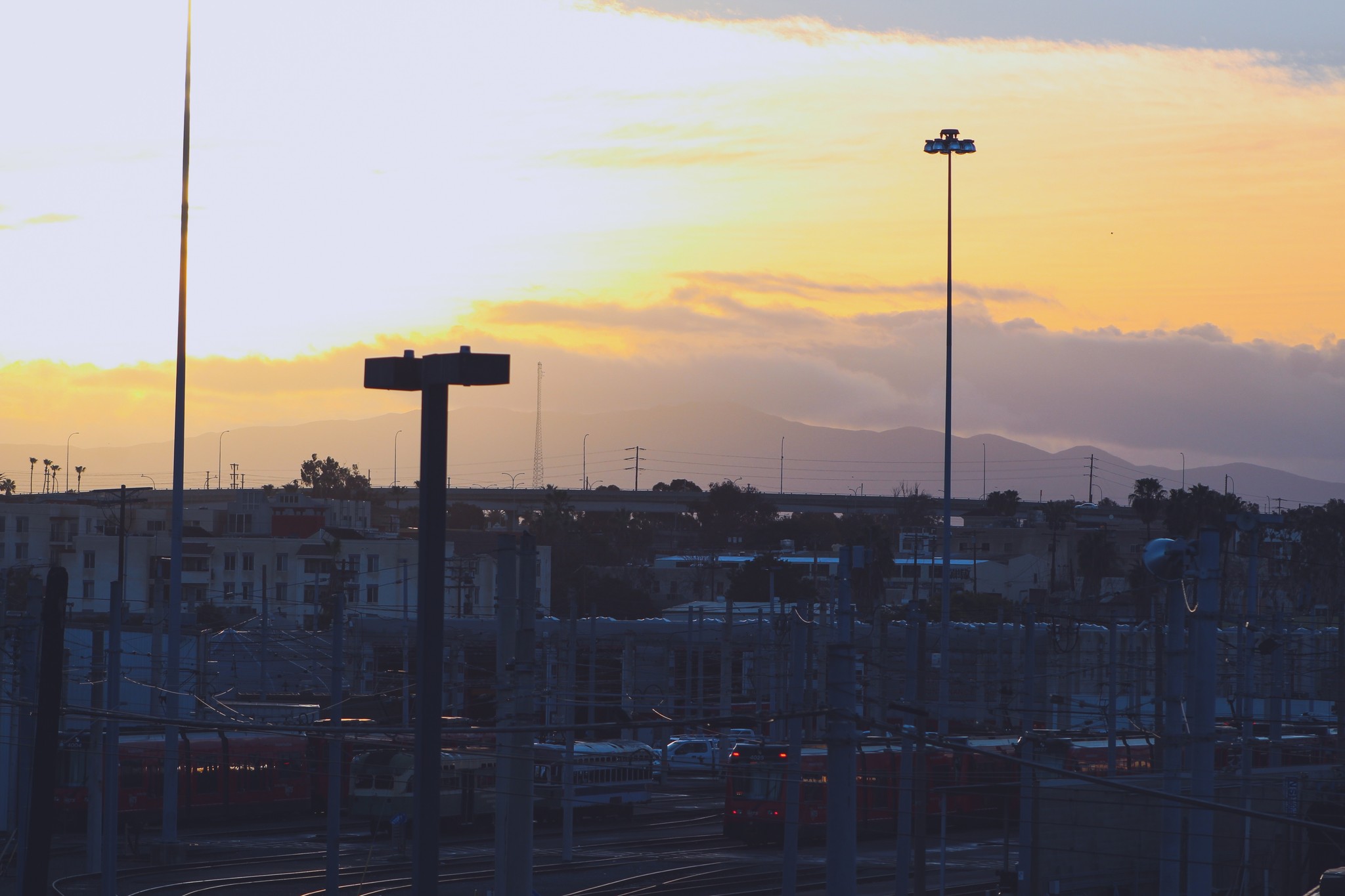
[58,716,1344,845]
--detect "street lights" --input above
[364,346,511,895]
[923,127,974,747]
